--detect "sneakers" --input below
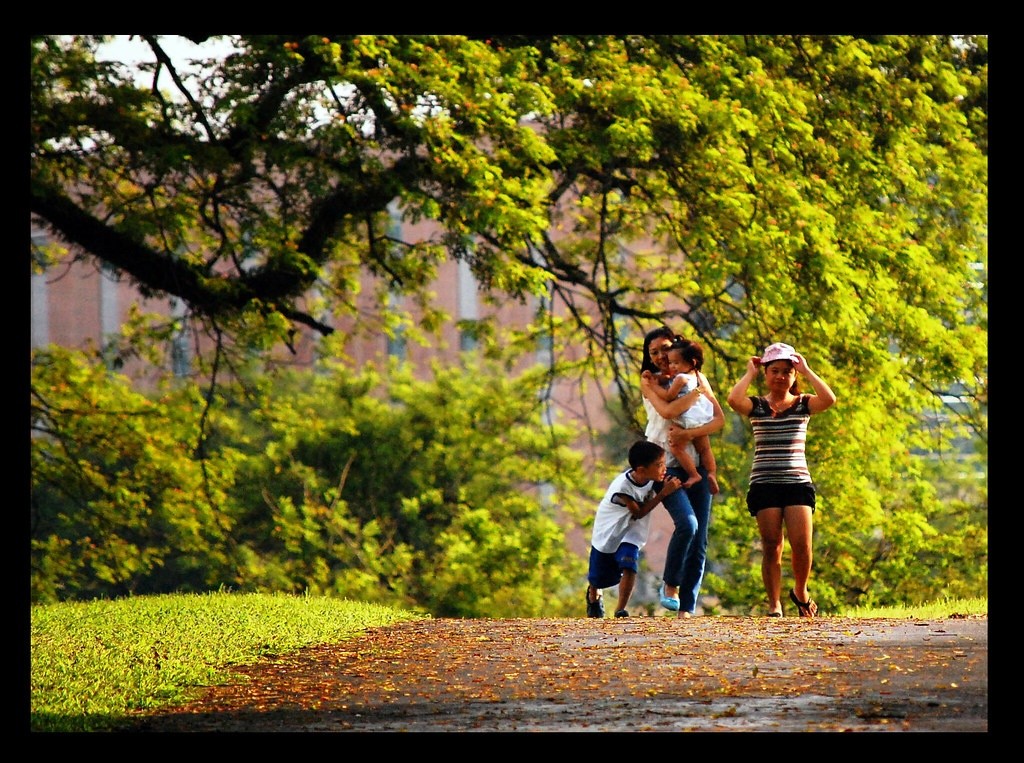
[614,609,629,617]
[586,586,604,618]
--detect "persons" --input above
[586,440,683,618]
[640,326,725,619]
[727,342,836,620]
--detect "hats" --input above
[760,343,799,364]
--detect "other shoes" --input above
[658,582,680,610]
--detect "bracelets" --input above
[805,369,814,381]
[692,388,701,398]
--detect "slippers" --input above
[767,612,781,617]
[789,589,819,617]
